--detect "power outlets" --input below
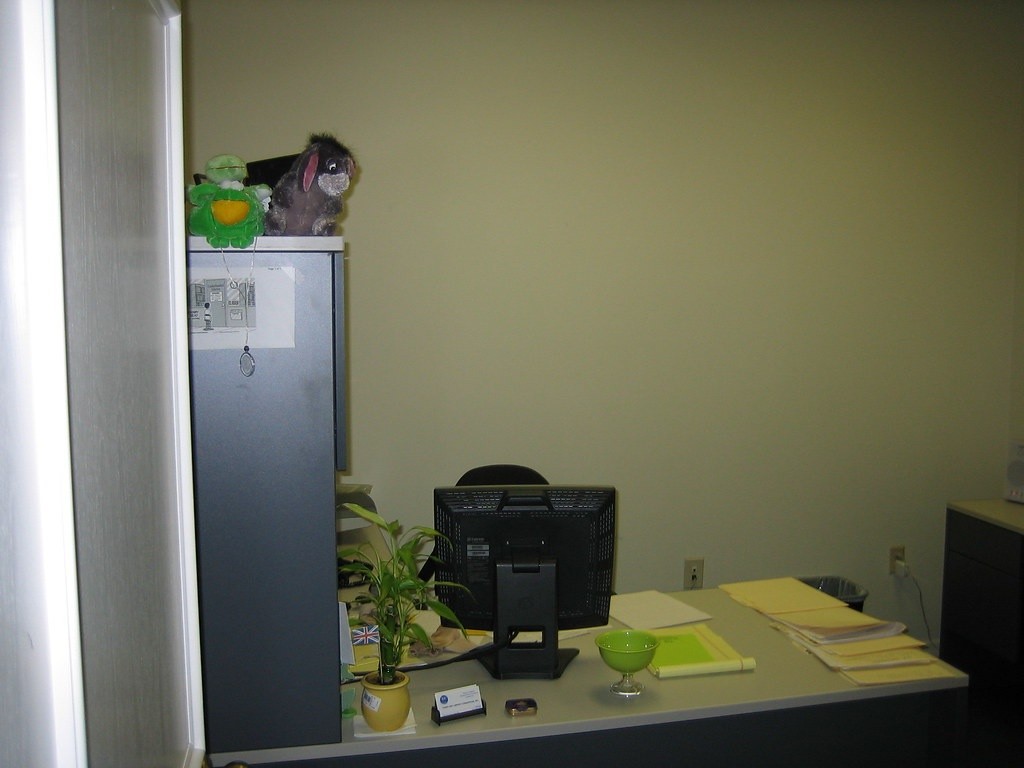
[889,545,905,575]
[685,558,704,590]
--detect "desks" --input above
[210,587,969,768]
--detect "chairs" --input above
[415,465,618,609]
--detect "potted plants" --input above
[337,503,476,732]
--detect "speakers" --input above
[1004,441,1024,503]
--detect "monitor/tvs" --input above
[432,484,615,680]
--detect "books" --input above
[645,622,756,680]
[770,623,954,687]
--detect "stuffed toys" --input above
[185,154,272,249]
[264,133,357,236]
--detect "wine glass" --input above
[594,628,661,695]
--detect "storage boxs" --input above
[336,517,400,584]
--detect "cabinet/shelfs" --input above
[940,499,1024,768]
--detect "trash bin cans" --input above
[797,574,868,612]
